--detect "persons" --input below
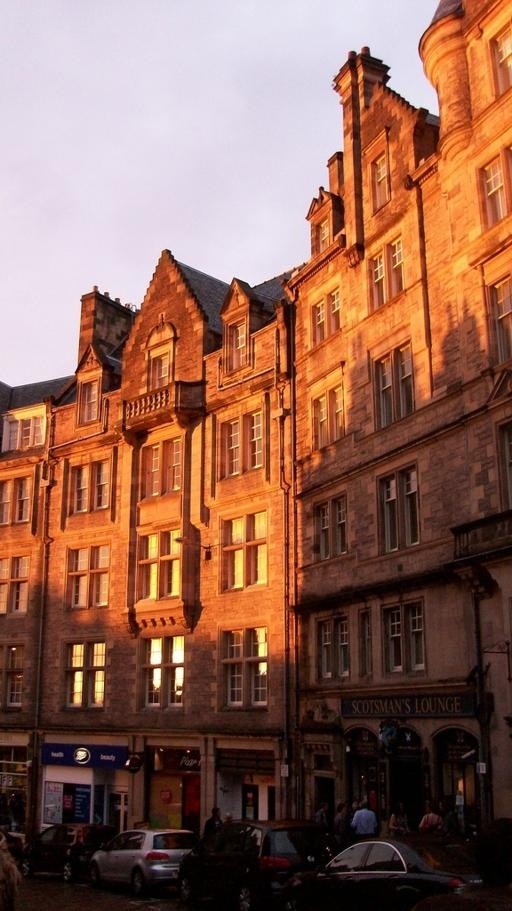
[1,797,512,910]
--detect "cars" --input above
[89,827,200,895]
[22,823,118,884]
[175,817,339,911]
[280,838,492,911]
